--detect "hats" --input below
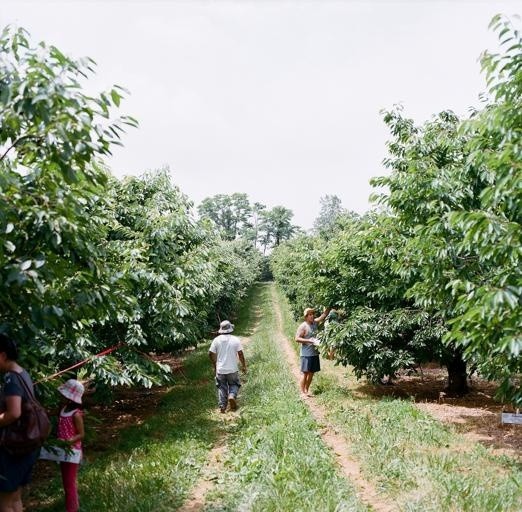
[218,319,235,334]
[57,378,85,404]
[302,308,315,321]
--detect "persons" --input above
[1,332,41,511]
[56,378,86,511]
[294,306,330,394]
[209,320,247,413]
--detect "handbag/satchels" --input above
[0,399,52,455]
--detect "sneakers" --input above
[219,395,236,414]
